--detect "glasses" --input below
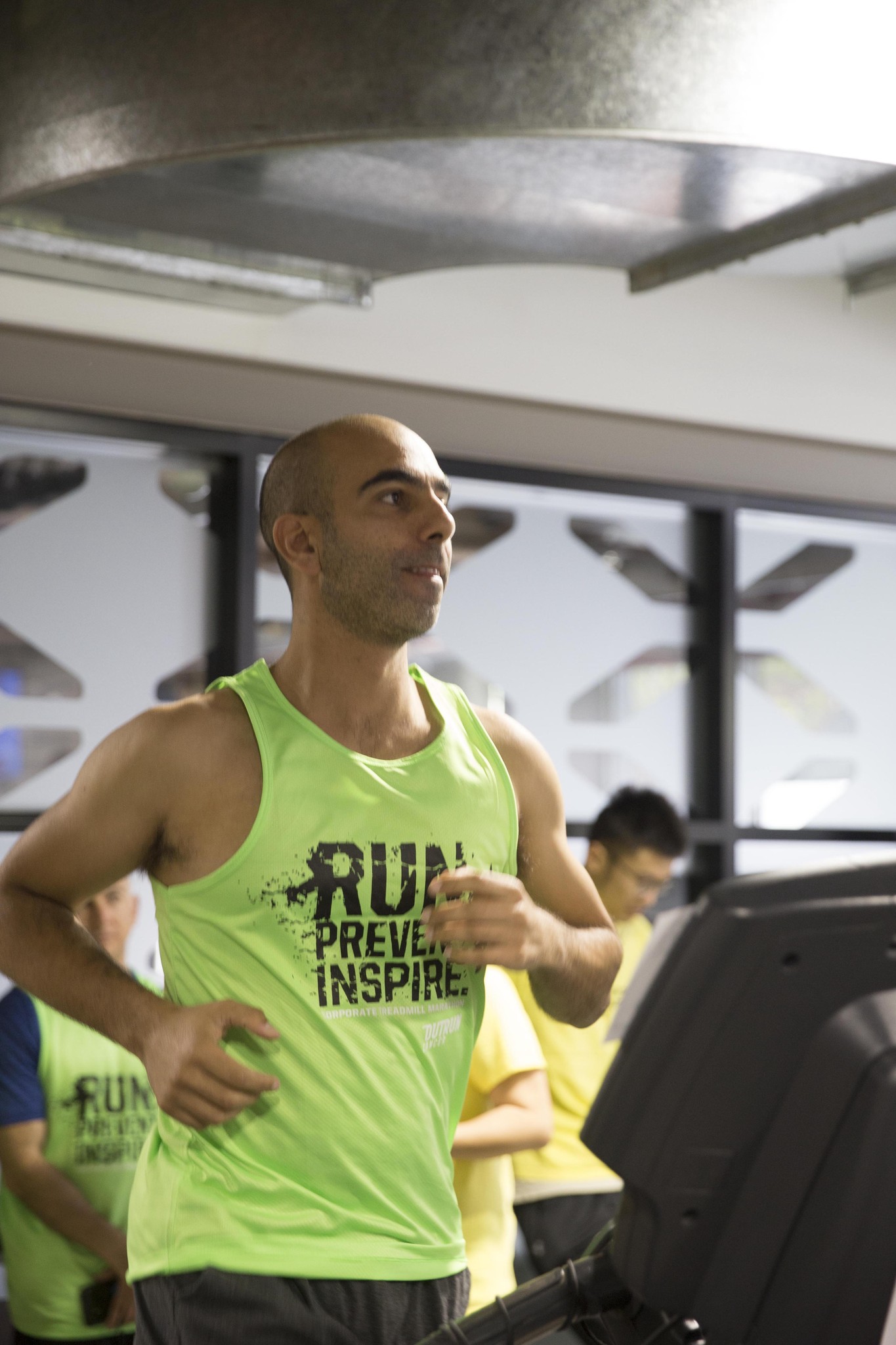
[627,874,669,890]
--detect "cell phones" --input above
[82,1277,119,1326]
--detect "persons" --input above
[0,866,170,1345]
[501,787,692,1270]
[0,415,622,1345]
[448,964,552,1317]
